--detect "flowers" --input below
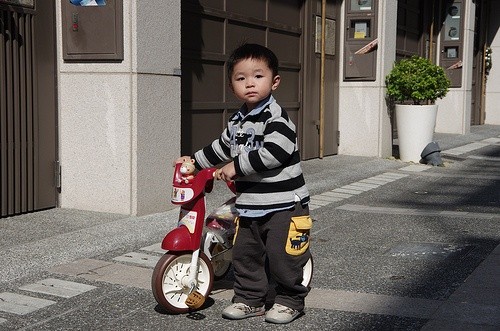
[485,47,493,75]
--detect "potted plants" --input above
[385,55,450,162]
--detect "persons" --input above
[176,43,314,323]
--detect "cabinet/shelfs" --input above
[439,0,466,86]
[342,0,378,81]
[61,0,124,62]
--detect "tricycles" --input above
[151,157,314,314]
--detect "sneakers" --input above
[264,301,301,324]
[222,303,265,319]
